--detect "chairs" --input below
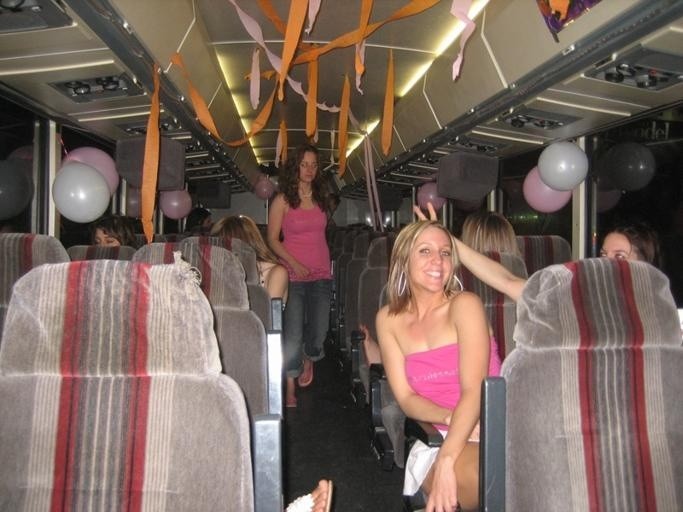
[327,222,394,421]
[64,241,135,264]
[3,247,258,512]
[124,239,286,512]
[477,254,683,511]
[2,231,75,307]
[177,234,285,427]
[373,248,529,472]
[513,232,573,282]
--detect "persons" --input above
[415,203,659,301]
[185,208,227,236]
[376,222,500,512]
[267,144,334,407]
[90,215,142,251]
[219,215,289,311]
[460,211,517,255]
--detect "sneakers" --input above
[285,395,298,408]
[298,356,314,388]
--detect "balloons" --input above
[417,182,445,211]
[0,146,33,222]
[538,141,588,190]
[606,143,656,192]
[159,189,192,221]
[52,160,111,224]
[598,170,621,213]
[63,145,120,196]
[257,174,280,198]
[523,166,572,213]
